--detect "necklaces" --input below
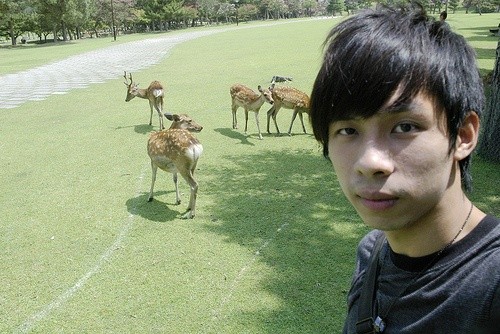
[371,200,474,334]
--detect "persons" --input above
[437,11,451,31]
[306,0,500,334]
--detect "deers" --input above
[229,84,275,141]
[146,112,204,219]
[266,86,311,137]
[123,70,166,131]
